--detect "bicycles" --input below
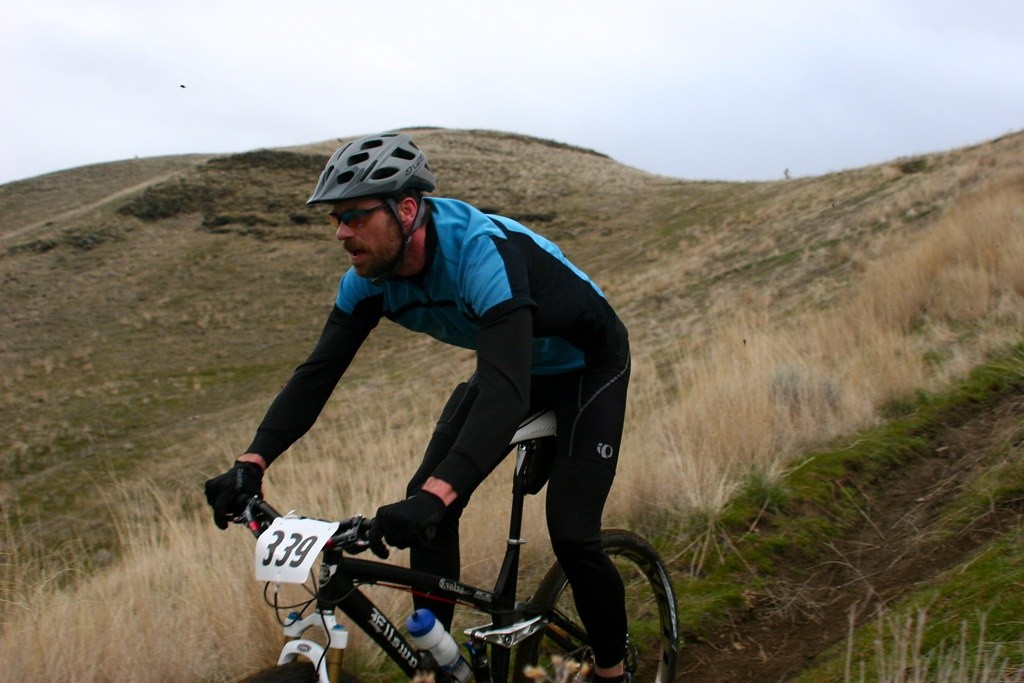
[229,488,680,682]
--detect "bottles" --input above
[406,608,475,683]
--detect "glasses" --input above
[323,199,405,228]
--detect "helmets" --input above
[306,134,436,204]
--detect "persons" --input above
[201,132,632,683]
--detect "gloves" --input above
[204,459,264,530]
[368,488,449,559]
